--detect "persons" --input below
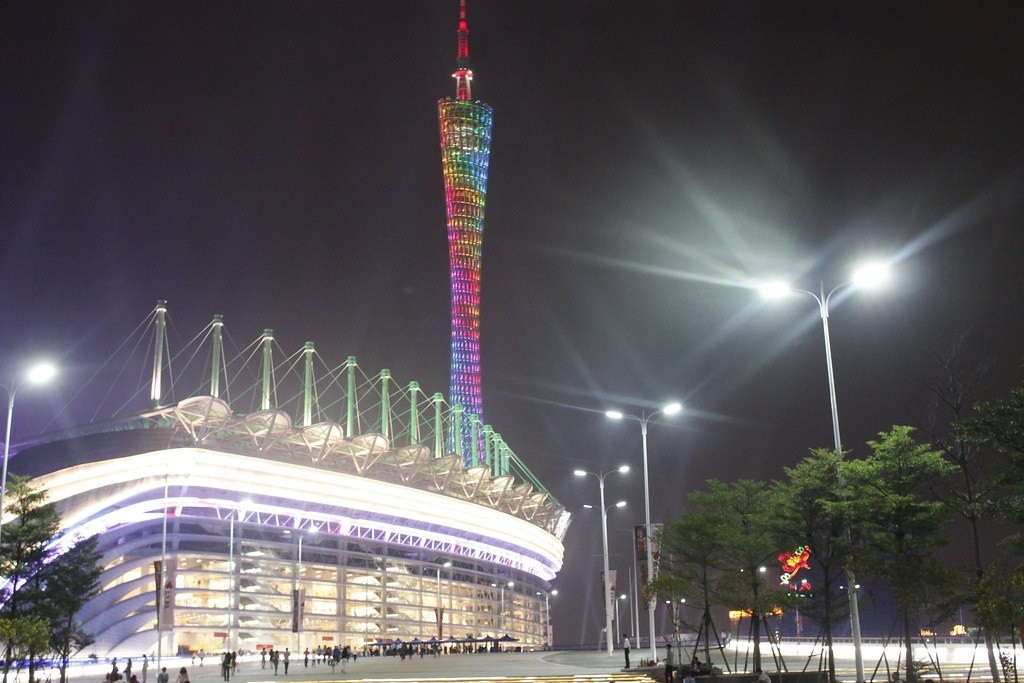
[691,657,703,678]
[260,648,290,676]
[892,671,907,683]
[755,668,771,683]
[622,634,632,669]
[721,630,731,648]
[221,651,237,681]
[105,654,190,683]
[303,644,358,674]
[600,573,605,582]
[361,642,487,661]
[151,650,155,662]
[635,527,648,559]
[191,648,205,665]
[663,644,674,683]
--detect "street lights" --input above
[1,359,55,517]
[574,466,629,656]
[283,526,320,657]
[605,402,683,663]
[437,562,451,640]
[580,501,626,653]
[492,581,514,651]
[839,586,861,637]
[756,259,893,683]
[783,580,808,638]
[228,499,253,656]
[536,590,559,649]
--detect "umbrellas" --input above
[389,636,519,652]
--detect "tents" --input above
[369,634,389,652]
